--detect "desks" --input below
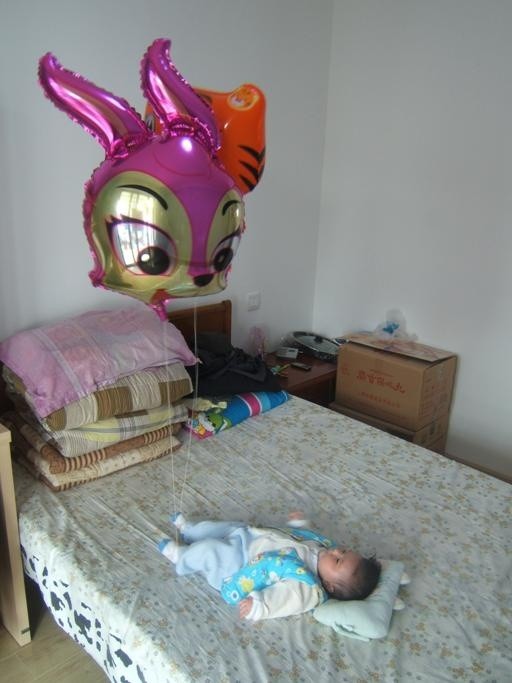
[0,422,32,647]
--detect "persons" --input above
[154,508,384,625]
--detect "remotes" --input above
[291,362,313,370]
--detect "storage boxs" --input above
[336,331,457,430]
[329,402,449,454]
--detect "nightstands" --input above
[255,348,336,405]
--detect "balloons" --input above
[140,80,272,202]
[33,38,244,323]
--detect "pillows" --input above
[0,305,203,418]
[314,559,409,641]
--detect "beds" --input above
[0,300,512,683]
